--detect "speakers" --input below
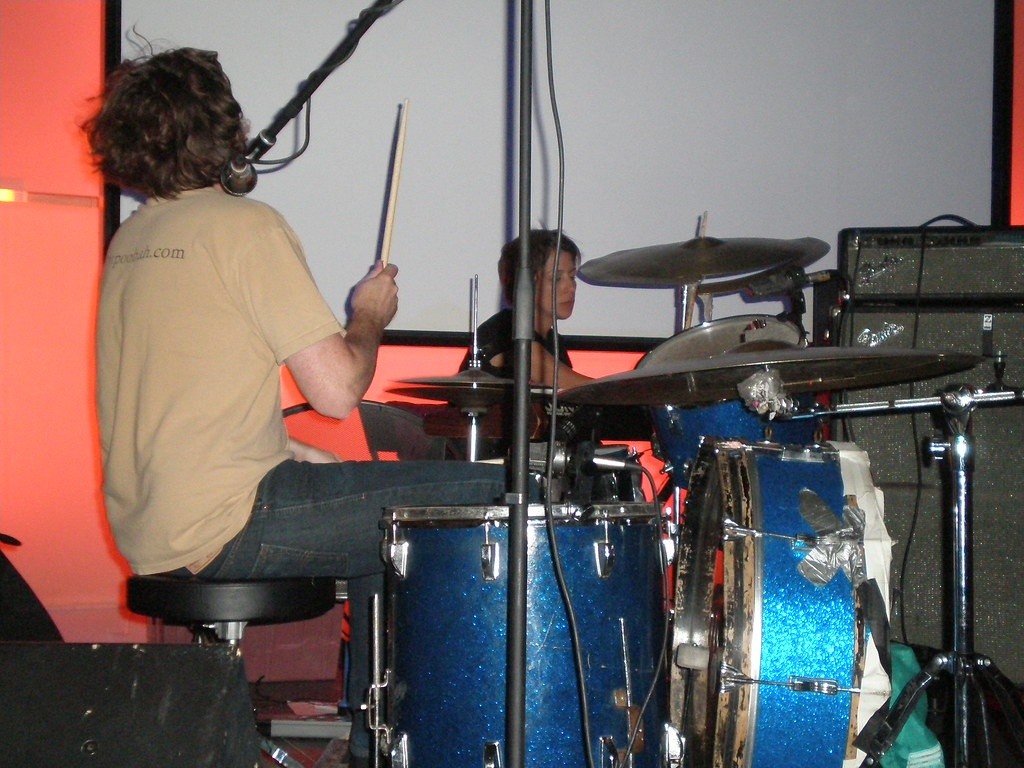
[0,641,266,768]
[831,305,1024,692]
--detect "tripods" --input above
[744,373,1023,768]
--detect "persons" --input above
[458,227,653,441]
[83,22,539,768]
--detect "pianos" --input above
[830,224,1024,690]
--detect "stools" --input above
[125,573,339,768]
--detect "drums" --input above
[635,314,823,491]
[670,435,867,768]
[472,444,635,505]
[378,500,666,768]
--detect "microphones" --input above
[507,441,642,474]
[754,269,838,296]
[219,154,257,196]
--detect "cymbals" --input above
[576,237,831,290]
[556,346,986,407]
[384,370,554,405]
